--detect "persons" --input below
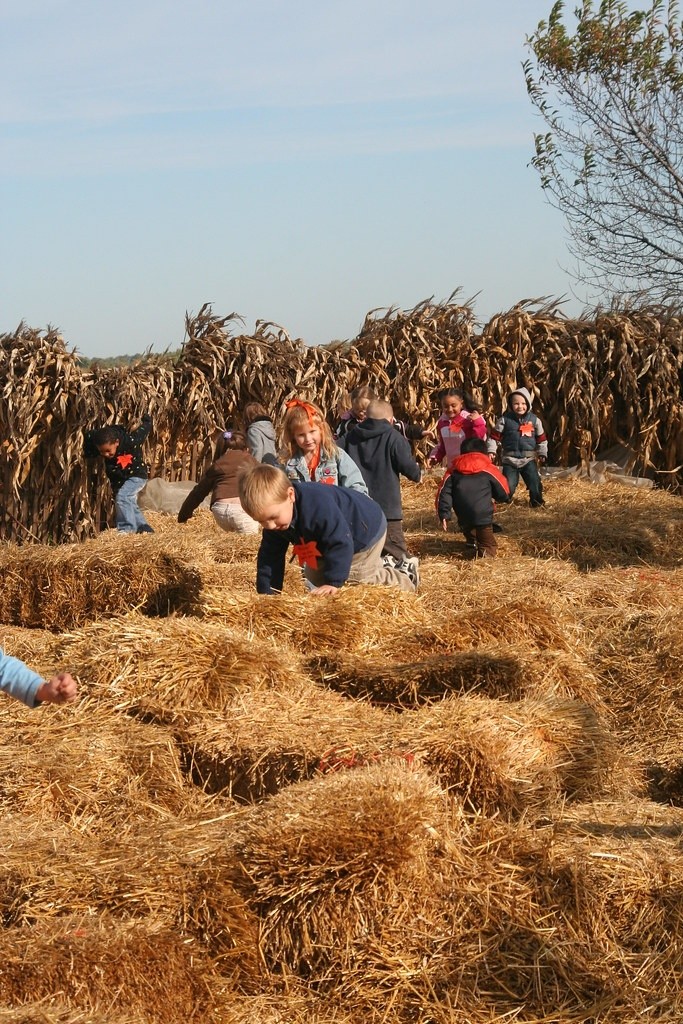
[237,462,420,598]
[0,646,78,710]
[87,413,157,534]
[178,387,548,566]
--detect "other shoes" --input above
[383,554,398,569]
[493,522,503,533]
[394,554,420,592]
